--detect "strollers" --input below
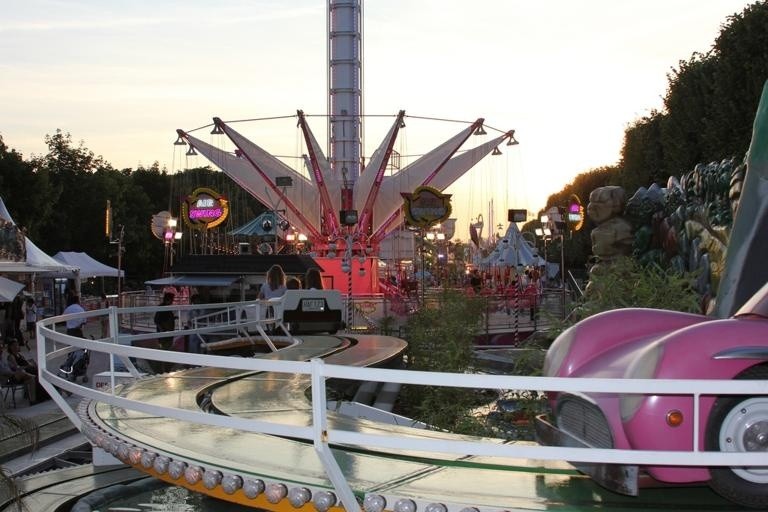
[57,335,96,384]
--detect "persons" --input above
[470,268,485,295]
[0,290,207,401]
[259,264,288,299]
[306,267,322,290]
[286,278,302,291]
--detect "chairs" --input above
[1,375,29,408]
